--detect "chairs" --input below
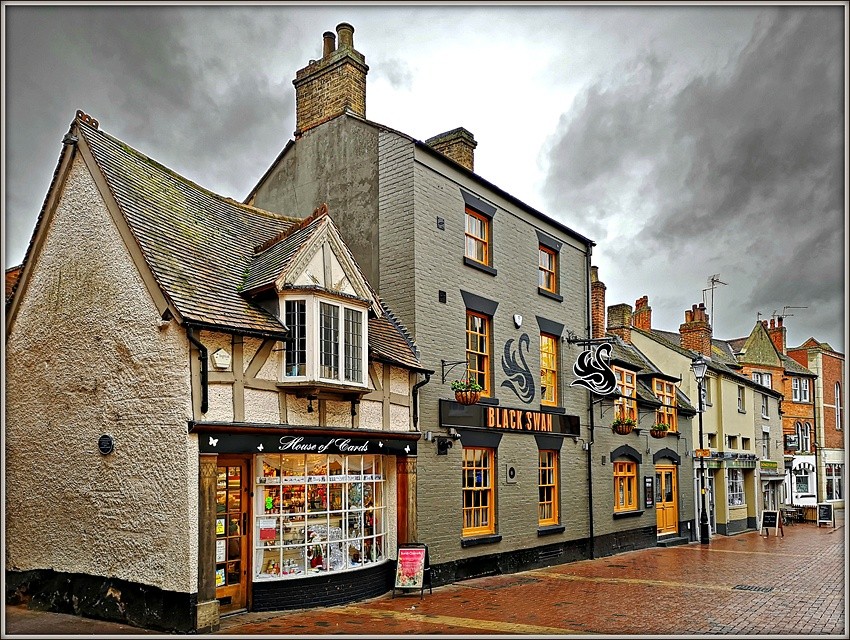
[780,507,810,527]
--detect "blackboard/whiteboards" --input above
[761,509,781,529]
[816,503,834,522]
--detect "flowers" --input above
[450,377,483,392]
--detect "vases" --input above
[454,389,481,405]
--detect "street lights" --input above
[691,351,709,545]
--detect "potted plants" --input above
[610,416,638,436]
[650,421,670,439]
